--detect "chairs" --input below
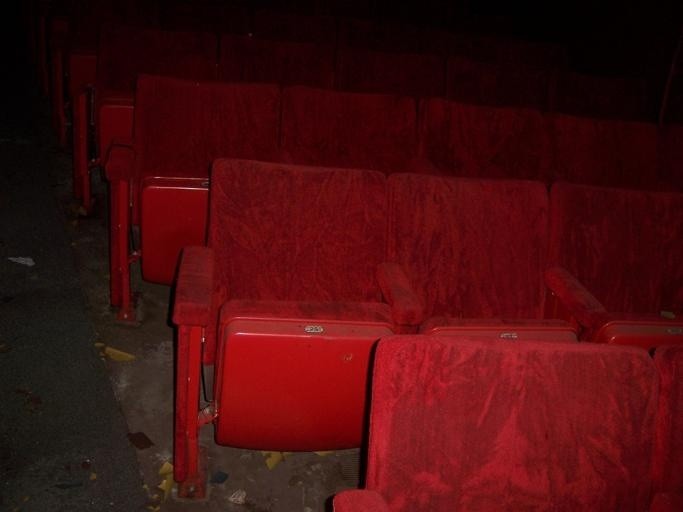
[21,0,683,512]
